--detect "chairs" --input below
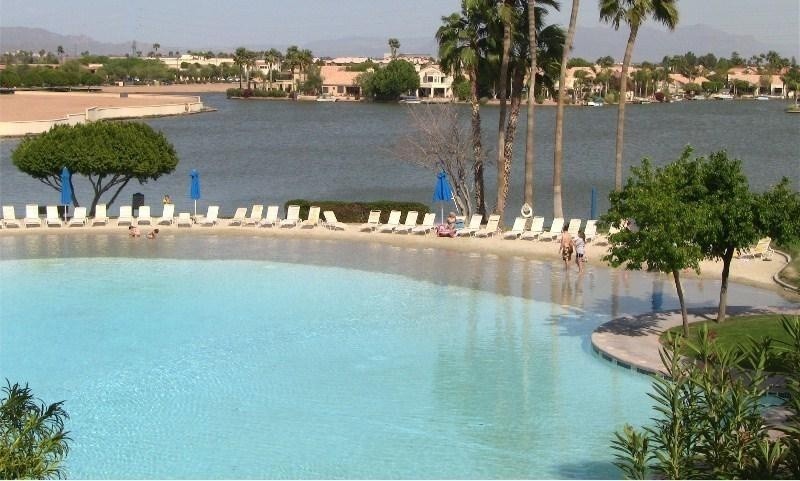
[732,236,774,260]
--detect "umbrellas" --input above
[432,165,452,224]
[60,167,73,224]
[189,168,201,216]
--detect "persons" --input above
[559,226,572,271]
[163,195,171,206]
[573,230,585,274]
[622,217,633,231]
[147,229,159,239]
[128,226,140,239]
[448,212,459,238]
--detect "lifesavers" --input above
[521,205,532,218]
